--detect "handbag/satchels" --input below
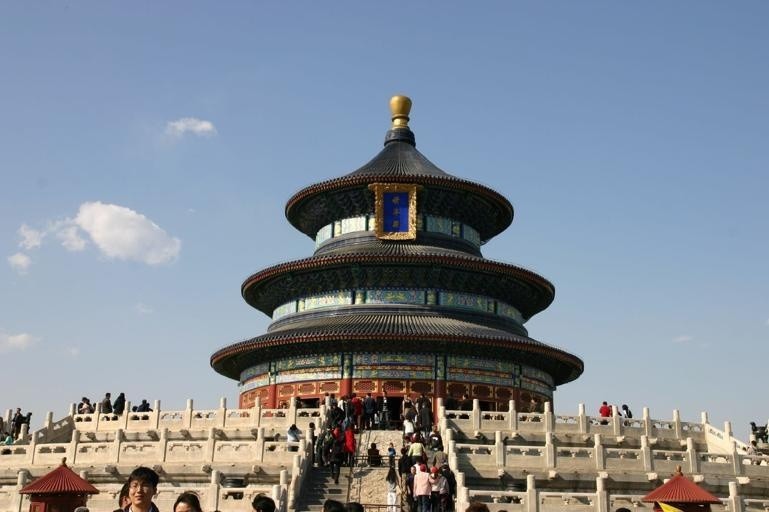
[422,452,428,462]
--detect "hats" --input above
[432,467,437,472]
[420,464,426,470]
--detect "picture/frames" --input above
[370,183,424,240]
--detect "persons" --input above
[599,402,610,425]
[615,508,632,512]
[0,431,12,445]
[250,492,276,512]
[622,405,632,427]
[172,490,204,512]
[76,392,153,421]
[74,506,89,512]
[122,466,161,512]
[314,389,509,512]
[11,407,23,440]
[747,440,761,465]
[113,483,133,512]
[610,405,622,416]
[22,411,32,434]
